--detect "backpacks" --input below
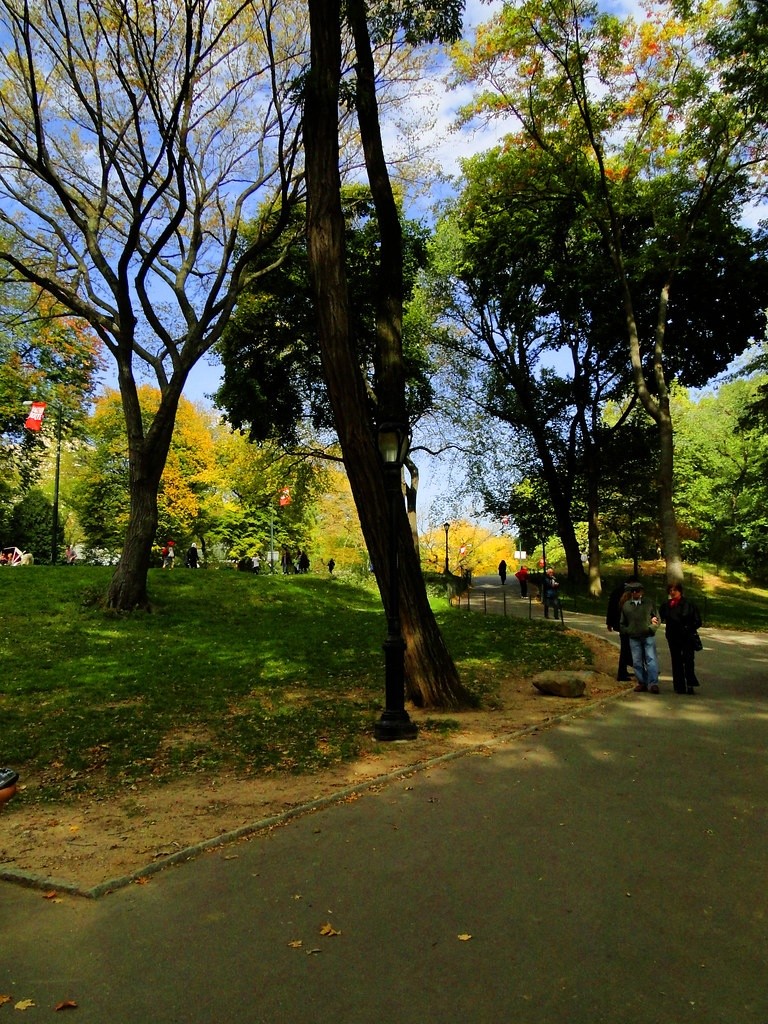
[162,547,169,555]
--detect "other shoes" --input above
[687,687,694,695]
[625,673,635,676]
[635,684,648,692]
[617,677,631,681]
[651,685,659,694]
[555,617,559,619]
[677,687,686,694]
[545,616,549,619]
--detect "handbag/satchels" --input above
[691,633,703,651]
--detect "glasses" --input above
[630,589,641,593]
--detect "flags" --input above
[278,486,292,505]
[25,401,46,430]
[502,514,512,526]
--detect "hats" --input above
[630,583,644,588]
[168,541,174,547]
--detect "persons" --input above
[543,568,560,620]
[161,540,336,574]
[498,560,506,584]
[581,553,587,564]
[606,575,703,696]
[0,553,20,567]
[537,557,545,569]
[515,566,530,599]
[64,545,77,566]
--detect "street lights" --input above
[23,400,63,565]
[443,522,450,572]
